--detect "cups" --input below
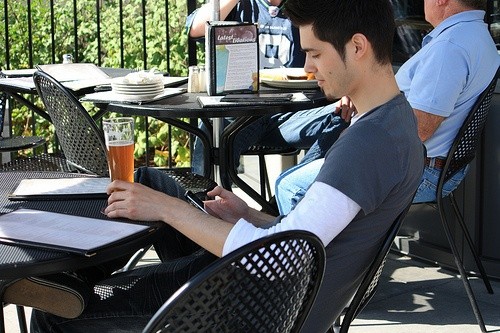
[102,117,135,194]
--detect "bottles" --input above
[187,65,200,92]
[197,63,207,93]
[62,54,72,63]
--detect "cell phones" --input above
[184,190,223,221]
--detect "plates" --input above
[259,68,320,88]
[109,76,165,96]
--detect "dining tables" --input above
[93,82,345,199]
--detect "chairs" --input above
[0,0,500,333]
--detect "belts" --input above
[424,156,443,169]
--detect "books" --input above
[9,178,116,199]
[0,208,157,259]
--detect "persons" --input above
[184,0,357,178]
[1,0,425,333]
[275,0,500,216]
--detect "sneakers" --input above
[0,271,93,320]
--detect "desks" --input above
[0,61,202,132]
[0,173,163,333]
